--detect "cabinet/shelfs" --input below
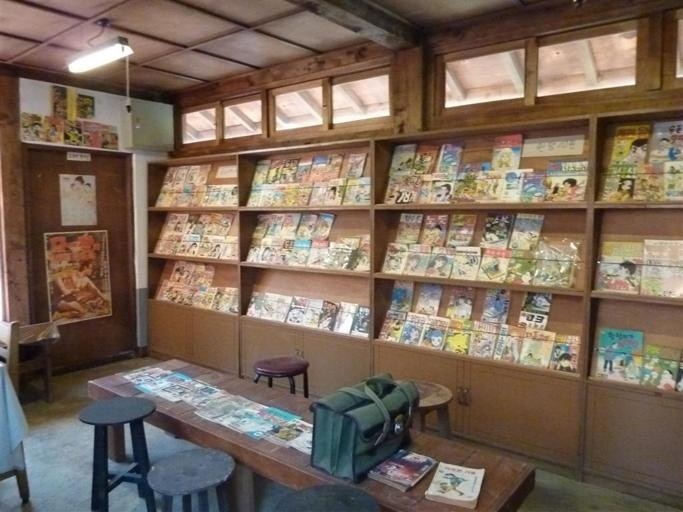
[148,105,683,509]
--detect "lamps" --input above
[67,18,134,73]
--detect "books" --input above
[155,164,237,316]
[384,134,588,203]
[124,367,313,456]
[425,462,485,510]
[244,154,371,338]
[368,449,436,492]
[379,280,581,372]
[596,121,681,392]
[381,213,578,289]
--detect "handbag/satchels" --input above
[308,372,418,481]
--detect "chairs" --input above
[0,321,54,502]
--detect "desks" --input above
[88,358,535,511]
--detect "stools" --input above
[252,358,309,400]
[276,485,382,512]
[145,447,235,512]
[395,378,453,439]
[79,397,155,512]
